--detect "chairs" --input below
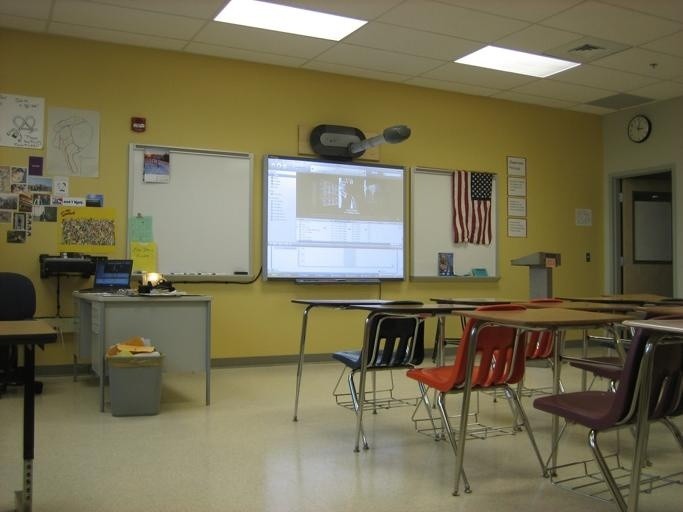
[0,271,45,397]
[533,311,681,510]
[521,299,564,396]
[569,298,683,466]
[332,300,440,452]
[405,303,550,497]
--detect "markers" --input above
[170,272,217,276]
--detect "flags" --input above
[453,170,492,245]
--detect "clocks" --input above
[626,115,651,145]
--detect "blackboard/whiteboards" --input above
[127,143,254,284]
[410,165,500,281]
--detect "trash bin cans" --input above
[106,352,166,417]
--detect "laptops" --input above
[80,260,134,294]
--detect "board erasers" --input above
[234,271,248,275]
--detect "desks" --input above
[290,296,613,423]
[73,290,214,413]
[450,309,682,510]
[0,320,58,511]
